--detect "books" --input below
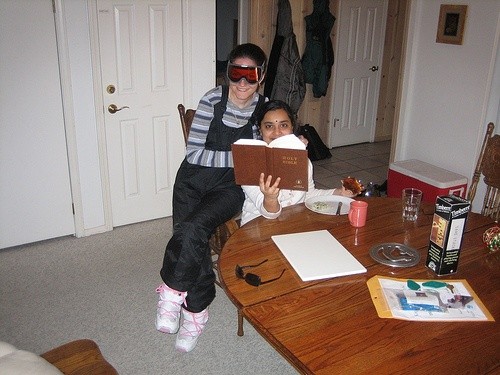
[230,134,308,192]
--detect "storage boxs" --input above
[425,196,470,276]
[387,158,468,205]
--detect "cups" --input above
[348,201,369,227]
[401,188,422,222]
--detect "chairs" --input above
[178,104,243,337]
[467,122,500,222]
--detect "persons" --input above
[155,44,269,353]
[239,101,362,227]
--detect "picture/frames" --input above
[435,3,469,45]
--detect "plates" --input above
[305,195,356,215]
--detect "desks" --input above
[218,196,500,375]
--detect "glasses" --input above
[235,259,287,286]
[227,60,266,85]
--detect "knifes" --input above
[336,201,342,215]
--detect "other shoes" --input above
[156,283,187,334]
[176,307,208,352]
